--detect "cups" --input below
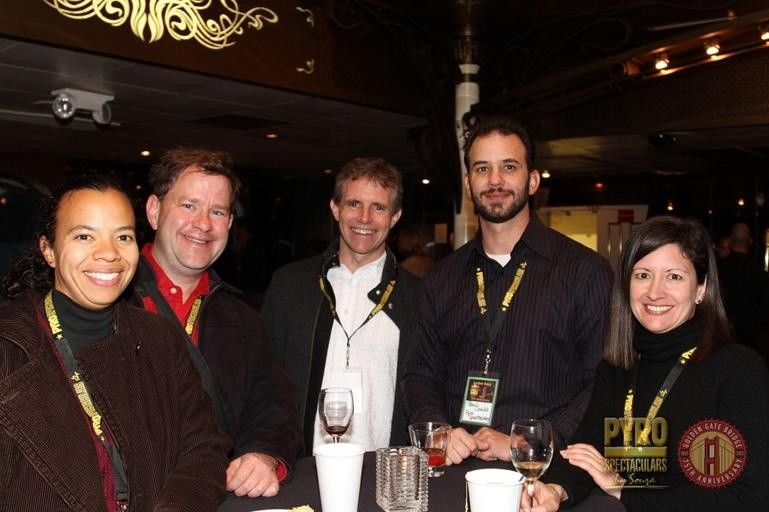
[310,442,366,512]
[374,445,430,512]
[464,468,528,512]
[407,421,453,480]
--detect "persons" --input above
[519,217,769,512]
[0,176,233,510]
[258,158,423,455]
[123,145,298,499]
[396,115,614,467]
[709,224,769,361]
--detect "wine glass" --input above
[315,386,356,442]
[508,418,555,511]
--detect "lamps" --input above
[52,88,115,124]
[652,53,670,70]
[702,36,721,56]
[612,57,645,77]
[760,27,769,41]
